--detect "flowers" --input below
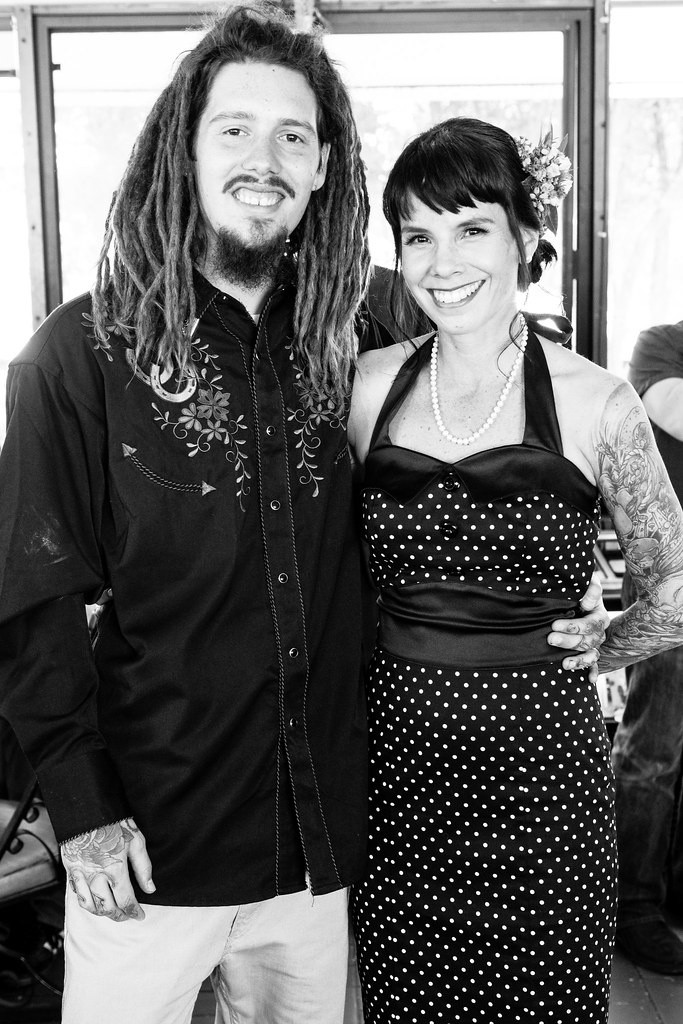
[513,118,578,237]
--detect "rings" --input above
[592,647,601,655]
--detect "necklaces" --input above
[430,314,528,445]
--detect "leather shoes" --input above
[616,918,683,974]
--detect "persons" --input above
[0,0,608,1024]
[347,118,683,1024]
[609,319,683,974]
